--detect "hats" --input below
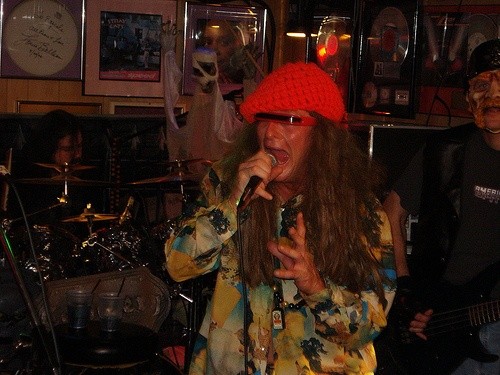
[239,62,345,125]
[468,39,500,80]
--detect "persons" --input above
[164,61,397,375]
[381,39,500,375]
[185,18,242,96]
[0,109,98,226]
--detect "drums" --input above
[19,223,79,274]
[149,214,185,285]
[93,223,149,273]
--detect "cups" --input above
[97,292,125,334]
[67,289,92,329]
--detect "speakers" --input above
[367,124,452,209]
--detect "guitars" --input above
[382,287,499,375]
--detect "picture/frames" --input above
[0,0,267,115]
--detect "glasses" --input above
[254,111,349,126]
[476,69,500,81]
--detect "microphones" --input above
[237,152,277,208]
[119,196,135,224]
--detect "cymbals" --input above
[125,156,206,185]
[16,161,113,187]
[61,212,120,222]
